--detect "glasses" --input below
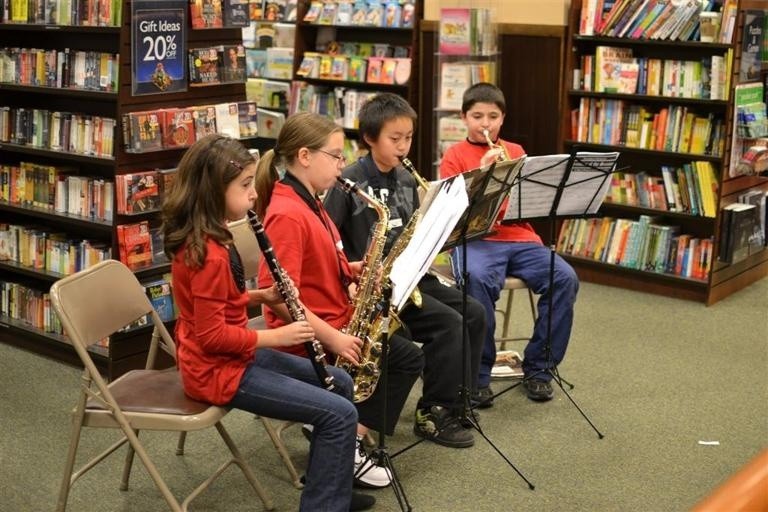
[307,146,345,170]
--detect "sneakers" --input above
[459,412,480,429]
[349,492,377,512]
[523,374,554,401]
[350,434,393,487]
[470,387,495,406]
[414,405,475,448]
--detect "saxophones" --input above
[334,176,420,402]
[382,155,430,314]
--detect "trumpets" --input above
[483,128,511,164]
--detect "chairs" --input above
[172,216,378,493]
[46,257,279,512]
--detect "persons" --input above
[255,114,424,488]
[440,82,580,406]
[161,134,376,512]
[323,94,487,447]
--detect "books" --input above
[245,0,500,169]
[0,0,261,351]
[557,0,768,280]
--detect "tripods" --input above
[353,300,412,512]
[389,237,535,491]
[472,211,603,438]
